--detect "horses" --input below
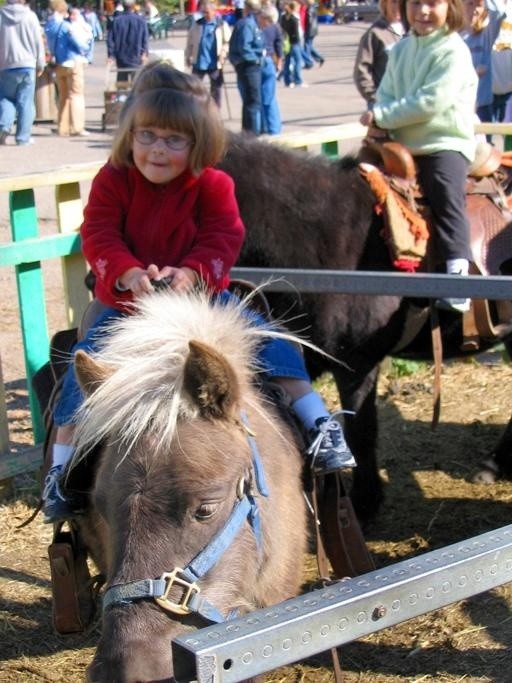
[219,133,512,531]
[37,272,353,683]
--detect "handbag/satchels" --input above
[49,56,56,65]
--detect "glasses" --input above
[132,130,195,150]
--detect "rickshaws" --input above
[102,62,145,132]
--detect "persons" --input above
[353,1,512,147]
[43,55,357,515]
[1,1,161,145]
[359,0,482,316]
[184,0,346,140]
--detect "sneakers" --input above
[435,297,471,314]
[310,415,357,477]
[72,129,90,135]
[43,464,86,523]
[0,129,34,146]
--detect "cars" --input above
[331,0,379,23]
